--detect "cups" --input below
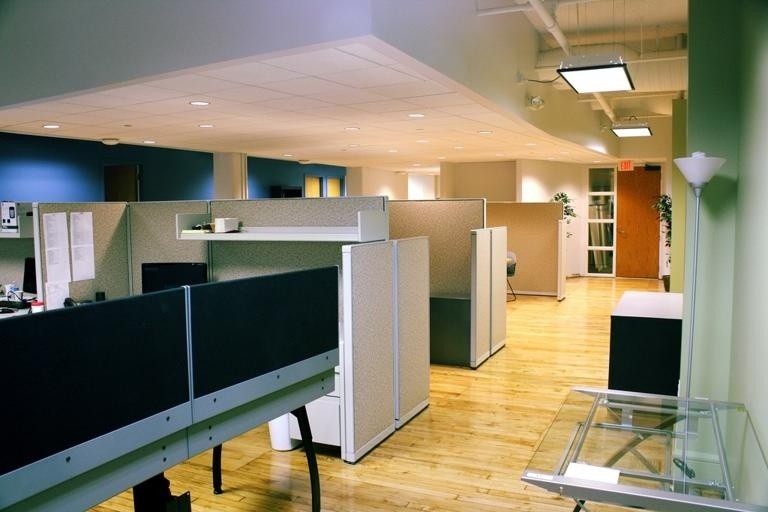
[14,291,23,302]
[30,302,44,314]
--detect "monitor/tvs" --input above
[23,258,36,294]
[141,262,206,294]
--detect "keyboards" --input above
[0,301,27,309]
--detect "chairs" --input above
[506,251,517,300]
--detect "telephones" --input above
[63,298,93,309]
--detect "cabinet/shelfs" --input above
[586,166,618,277]
[608,290,683,396]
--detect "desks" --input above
[390,198,510,370]
[0,193,433,466]
[521,386,768,511]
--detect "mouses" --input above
[0,308,13,313]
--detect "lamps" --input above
[611,116,653,138]
[557,2,636,94]
[671,150,727,398]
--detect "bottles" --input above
[10,280,19,292]
